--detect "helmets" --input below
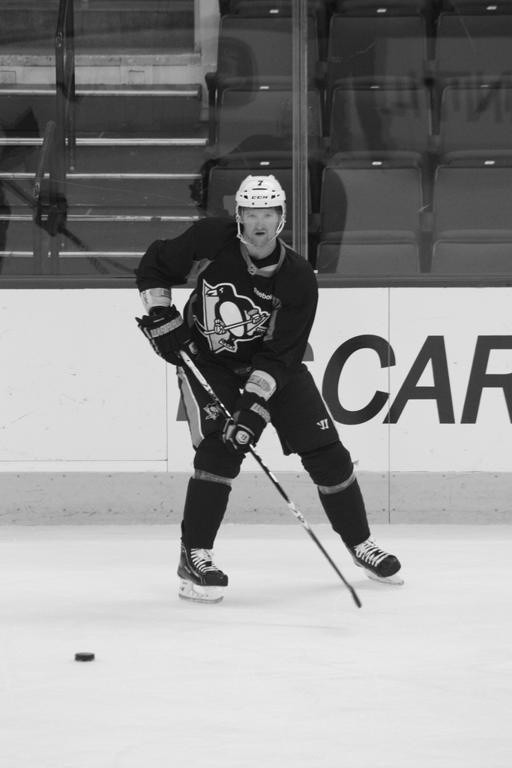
[234,174,288,224]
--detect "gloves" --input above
[135,303,201,367]
[217,391,272,457]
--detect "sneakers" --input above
[175,542,229,586]
[347,538,402,579]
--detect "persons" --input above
[134,173,402,589]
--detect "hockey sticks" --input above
[5,182,138,274]
[182,351,360,607]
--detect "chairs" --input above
[198,1,512,276]
